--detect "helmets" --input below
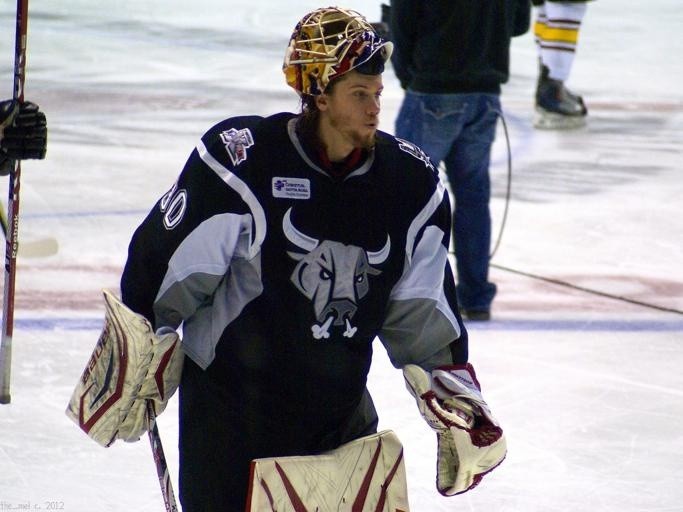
[280,7,395,99]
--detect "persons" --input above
[378,0,531,324]
[61,5,511,511]
[526,0,598,118]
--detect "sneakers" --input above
[454,298,491,321]
[533,57,590,120]
